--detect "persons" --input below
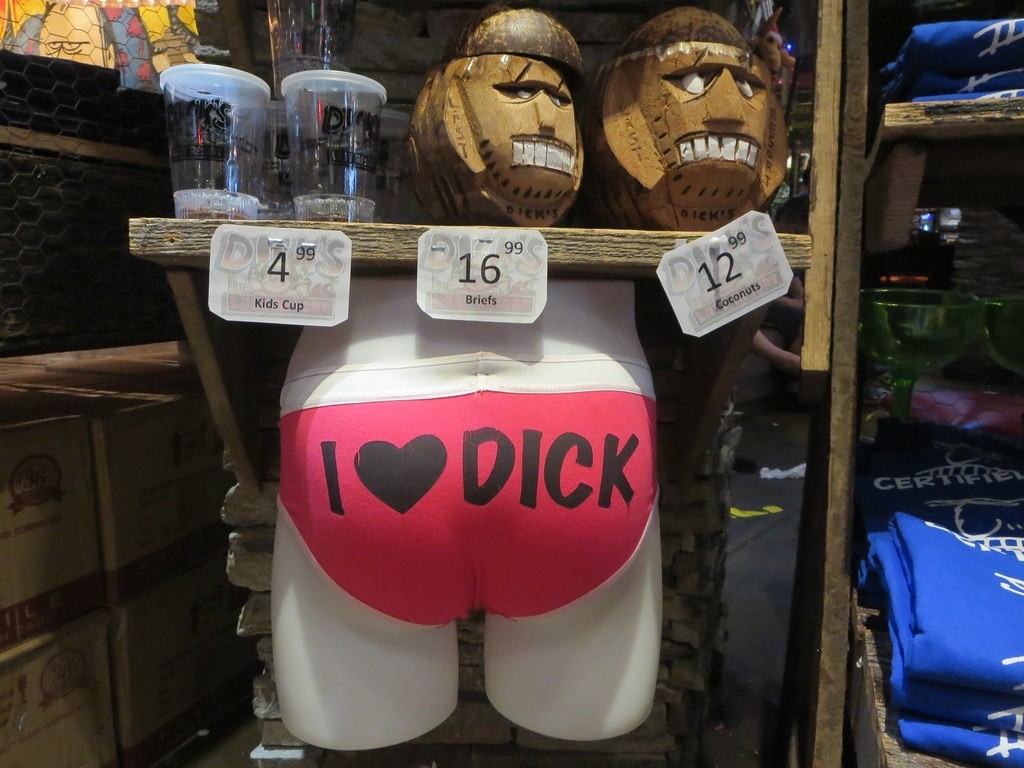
[727,194,819,417]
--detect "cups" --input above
[282,69,388,223]
[159,62,272,218]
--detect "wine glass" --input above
[860,280,1024,427]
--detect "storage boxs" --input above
[850,630,980,768]
[0,381,257,768]
